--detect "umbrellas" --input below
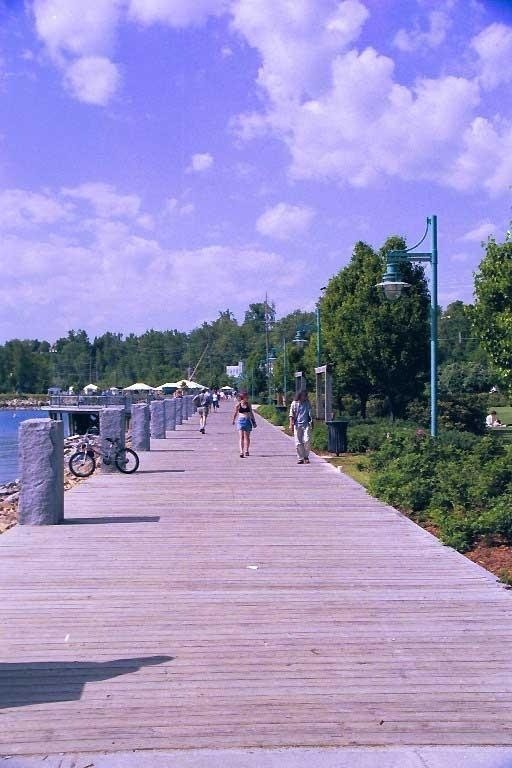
[80,379,210,395]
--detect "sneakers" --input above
[298,459,303,464]
[304,458,309,463]
[240,452,244,458]
[246,451,249,456]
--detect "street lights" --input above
[375,214,440,438]
[266,333,288,408]
[291,309,322,368]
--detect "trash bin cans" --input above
[276,407,287,413]
[327,421,349,453]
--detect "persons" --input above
[191,384,257,457]
[289,389,314,464]
[485,411,507,429]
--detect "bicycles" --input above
[69,424,139,476]
